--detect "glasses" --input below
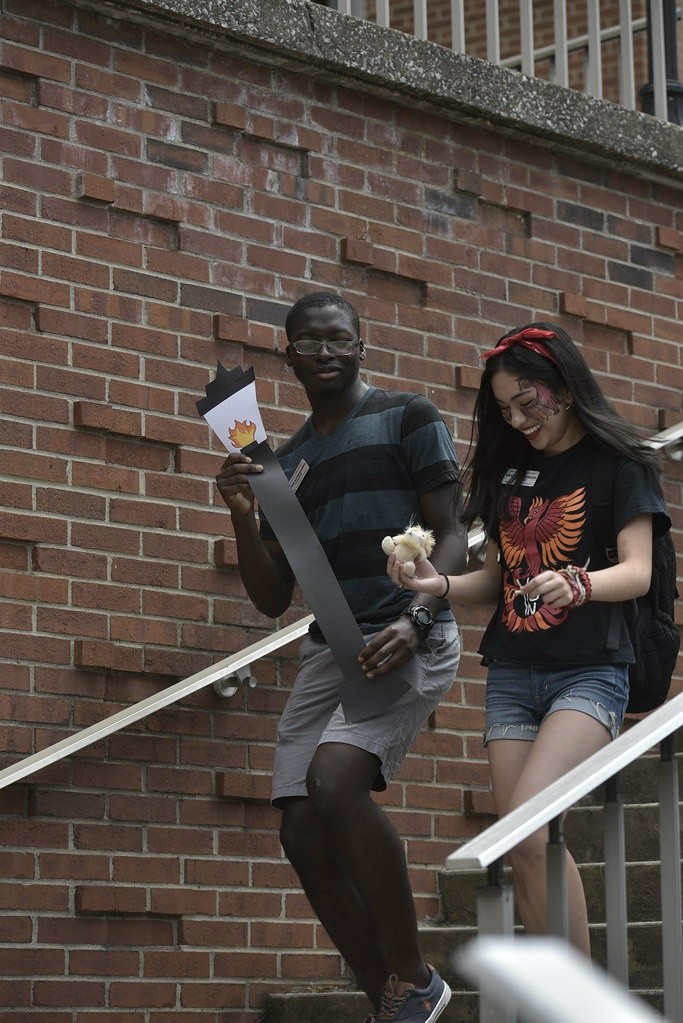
[287,340,364,356]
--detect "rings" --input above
[397,582,405,590]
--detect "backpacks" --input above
[599,449,679,715]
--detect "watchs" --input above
[399,605,435,639]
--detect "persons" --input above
[214,293,466,1023]
[384,322,674,963]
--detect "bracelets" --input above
[559,564,592,607]
[437,572,450,598]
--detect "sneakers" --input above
[363,963,452,1023]
